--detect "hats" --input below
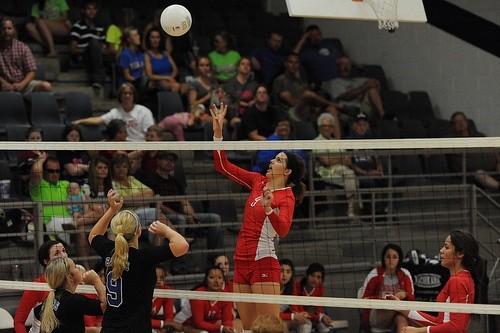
[355,112,368,122]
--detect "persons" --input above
[384,229,491,333]
[88,189,190,333]
[28,255,107,333]
[209,102,307,333]
[0,16,52,103]
[0,0,500,333]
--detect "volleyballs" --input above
[160,5,192,37]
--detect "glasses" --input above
[45,169,60,174]
